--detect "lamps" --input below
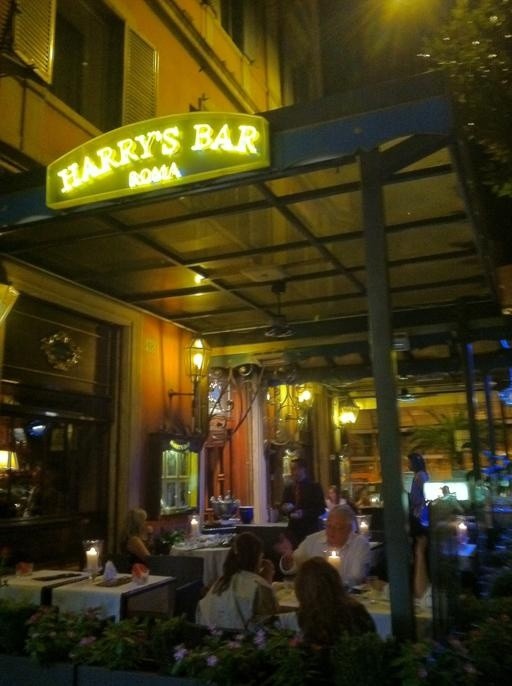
[166,334,216,398]
[262,281,297,338]
[0,450,19,499]
[395,349,417,402]
[284,378,317,425]
[329,393,362,430]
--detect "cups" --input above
[186,511,201,537]
[15,562,34,579]
[82,538,105,573]
[367,574,381,603]
[239,506,254,525]
[321,545,344,567]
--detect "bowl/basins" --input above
[212,500,238,523]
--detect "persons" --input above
[403,451,434,599]
[193,528,283,631]
[273,455,329,548]
[270,501,378,595]
[112,504,159,570]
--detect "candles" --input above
[459,521,468,537]
[328,551,341,570]
[358,521,369,534]
[85,547,99,571]
[190,519,200,535]
